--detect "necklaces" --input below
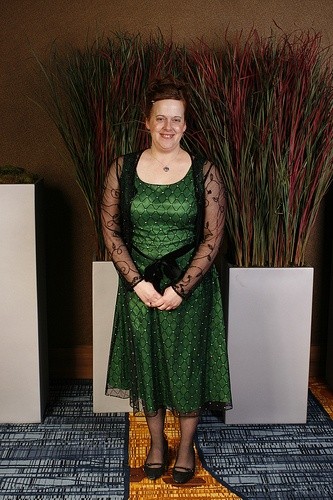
[151,149,180,173]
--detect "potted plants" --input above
[185,19,333,425]
[25,25,214,413]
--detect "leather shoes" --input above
[172,450,195,483]
[144,440,168,479]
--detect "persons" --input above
[102,77,233,484]
